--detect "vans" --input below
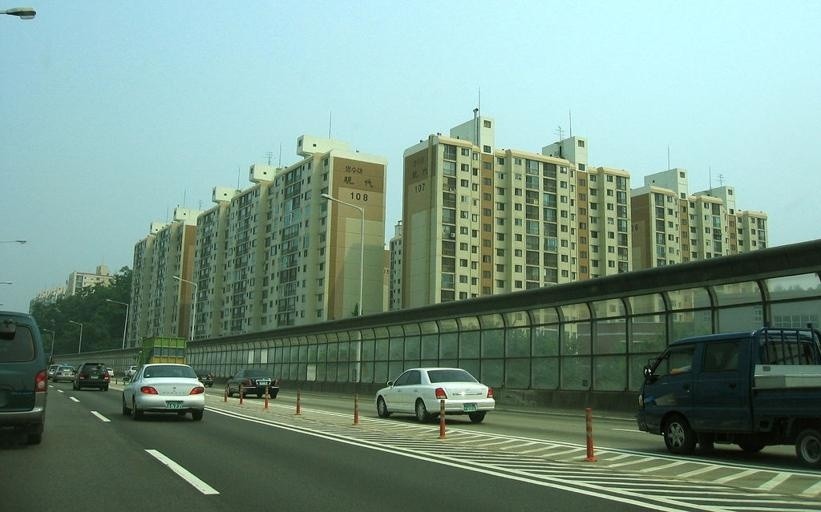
[1,310,48,446]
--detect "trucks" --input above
[636,323,821,468]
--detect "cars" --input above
[48,362,115,390]
[122,363,216,420]
[376,367,497,424]
[225,368,280,398]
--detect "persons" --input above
[670,365,691,374]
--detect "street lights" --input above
[106,298,130,348]
[43,328,56,362]
[319,192,365,383]
[172,275,199,342]
[69,319,84,352]
